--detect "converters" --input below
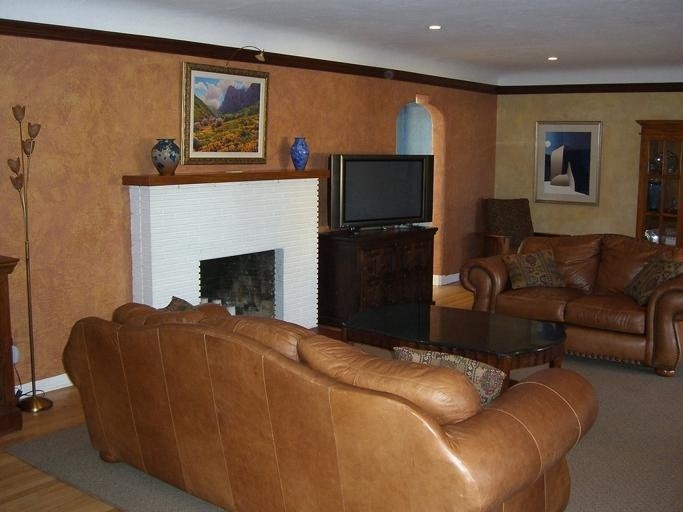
[16,390,22,400]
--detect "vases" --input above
[151,139,181,176]
[290,137,309,170]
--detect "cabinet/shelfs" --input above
[635,120,683,247]
[319,227,439,327]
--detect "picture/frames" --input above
[533,120,602,206]
[180,61,269,165]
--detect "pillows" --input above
[156,295,197,313]
[501,252,565,289]
[391,346,503,409]
[624,255,683,305]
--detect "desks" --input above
[0,256,23,437]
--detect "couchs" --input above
[63,306,599,512]
[460,234,683,377]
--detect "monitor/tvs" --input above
[326,153,435,232]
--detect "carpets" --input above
[5,356,683,512]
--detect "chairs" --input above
[481,197,571,254]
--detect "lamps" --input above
[226,46,266,65]
[7,104,53,413]
